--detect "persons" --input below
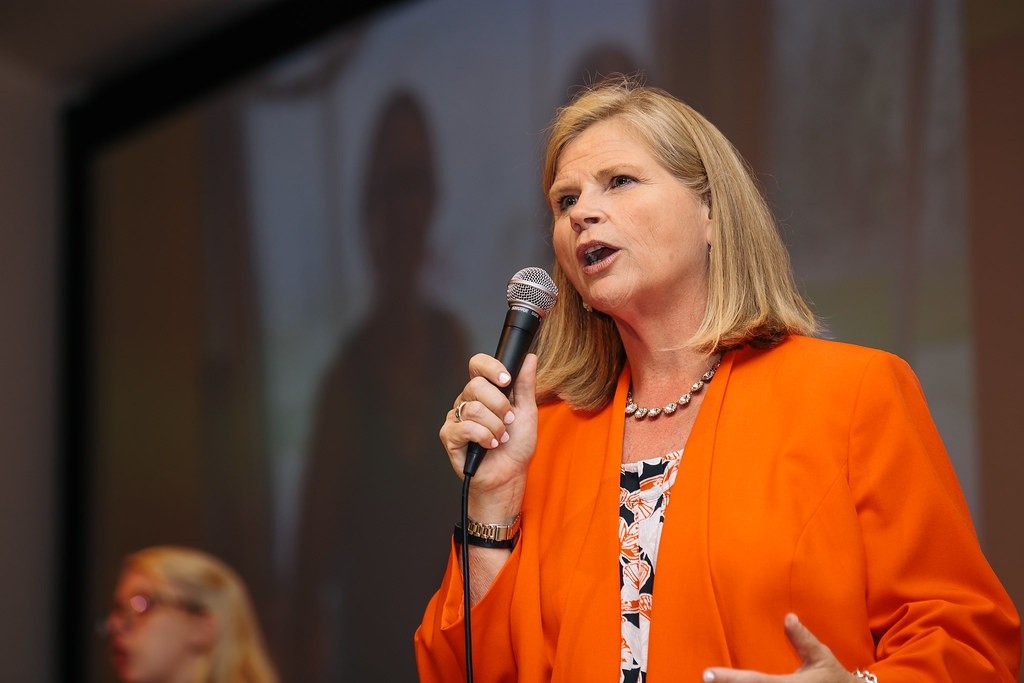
[415,87,1024,683]
[107,550,276,683]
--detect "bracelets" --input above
[852,669,878,683]
[454,521,514,551]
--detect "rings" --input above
[455,402,466,422]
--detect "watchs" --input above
[467,514,522,540]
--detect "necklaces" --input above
[625,360,720,420]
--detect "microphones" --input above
[461,267,558,477]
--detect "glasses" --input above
[106,593,204,616]
